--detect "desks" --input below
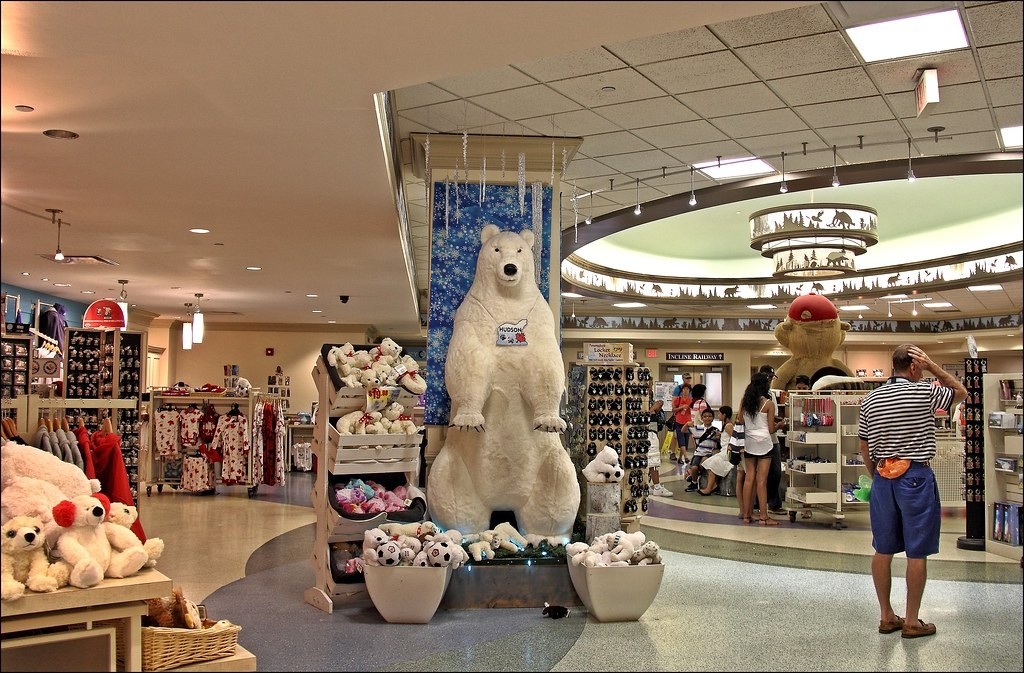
[1,568,172,672]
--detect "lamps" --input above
[586,139,917,226]
[858,302,917,319]
[45,208,64,260]
[571,302,575,318]
[118,280,128,330]
[746,205,880,281]
[183,294,203,350]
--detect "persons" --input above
[858,343,968,638]
[647,378,673,496]
[796,375,810,395]
[697,406,735,496]
[672,385,693,464]
[681,409,720,492]
[729,365,788,525]
[689,384,708,447]
[670,372,693,460]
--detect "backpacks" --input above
[658,409,666,431]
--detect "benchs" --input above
[700,464,736,496]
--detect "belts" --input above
[911,461,930,468]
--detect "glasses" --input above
[796,376,808,379]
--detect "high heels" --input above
[697,485,719,496]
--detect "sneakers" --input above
[687,475,692,482]
[653,485,673,496]
[879,615,906,634]
[685,483,698,491]
[901,619,936,637]
[768,507,787,514]
[753,505,760,511]
[670,453,677,460]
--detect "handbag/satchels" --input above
[800,397,834,427]
[666,416,676,431]
[727,449,741,465]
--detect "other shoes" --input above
[678,460,684,464]
[686,460,689,463]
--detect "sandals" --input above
[743,516,753,523]
[758,517,783,525]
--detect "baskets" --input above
[67,617,243,672]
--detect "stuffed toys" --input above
[770,292,855,391]
[565,529,662,567]
[1,441,165,602]
[236,376,252,391]
[333,478,528,573]
[148,590,230,630]
[327,337,427,450]
[427,225,582,548]
[582,446,619,483]
[542,601,571,619]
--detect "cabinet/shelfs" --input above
[982,372,1024,560]
[788,390,870,529]
[303,344,424,614]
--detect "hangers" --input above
[0,397,114,439]
[184,450,207,459]
[254,393,286,409]
[294,436,311,448]
[159,401,245,417]
[35,336,63,358]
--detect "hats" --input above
[760,365,778,378]
[682,372,692,378]
